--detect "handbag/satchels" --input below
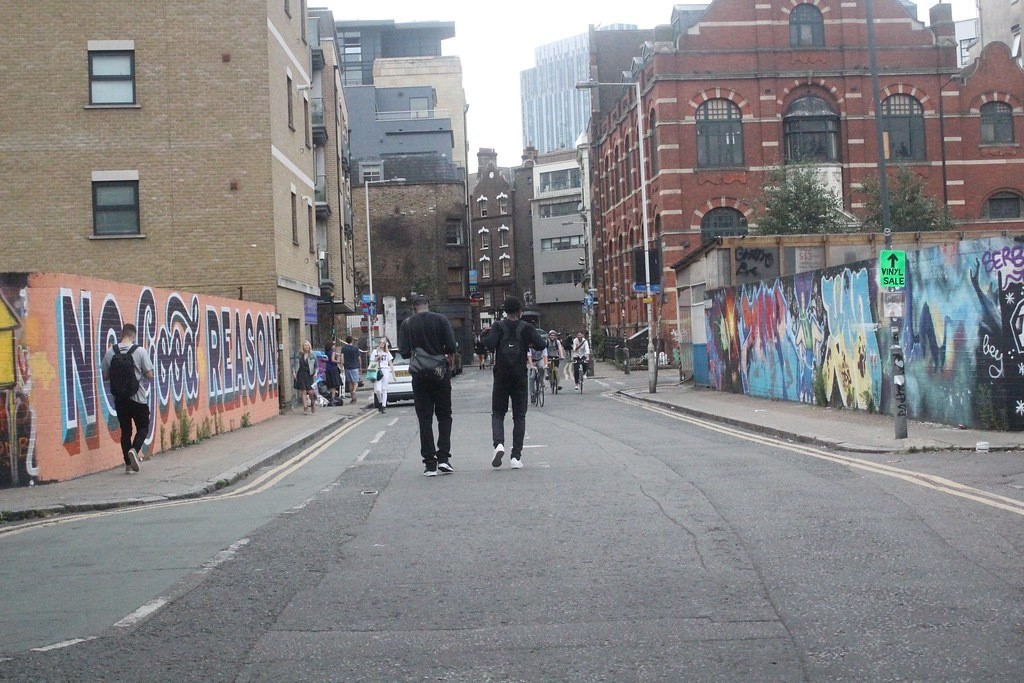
[366,361,378,370]
[366,370,377,379]
[408,347,447,382]
[377,370,383,380]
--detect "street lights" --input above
[574,79,657,397]
[563,221,595,377]
[936,73,960,228]
[365,177,405,354]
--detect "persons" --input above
[370,338,396,413]
[530,329,590,403]
[341,335,362,403]
[101,323,153,474]
[480,297,547,469]
[325,341,343,399]
[475,334,496,370]
[294,341,318,413]
[400,296,455,475]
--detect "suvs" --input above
[445,342,463,377]
[373,348,415,408]
[354,337,395,366]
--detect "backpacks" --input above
[499,319,527,378]
[109,344,142,397]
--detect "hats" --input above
[549,329,556,335]
[413,294,430,311]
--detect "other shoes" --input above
[583,375,587,379]
[382,407,386,412]
[129,448,140,472]
[349,391,356,405]
[311,401,315,412]
[304,405,307,414]
[558,385,562,390]
[541,382,545,387]
[126,465,133,473]
[531,396,535,403]
[545,375,549,380]
[574,386,578,391]
[480,363,482,369]
[482,364,484,370]
[379,403,382,411]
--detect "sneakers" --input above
[423,468,437,476]
[492,444,504,467]
[438,461,453,472]
[510,457,524,469]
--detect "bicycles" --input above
[571,356,589,393]
[530,367,550,407]
[546,358,565,394]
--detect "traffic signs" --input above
[879,248,907,290]
